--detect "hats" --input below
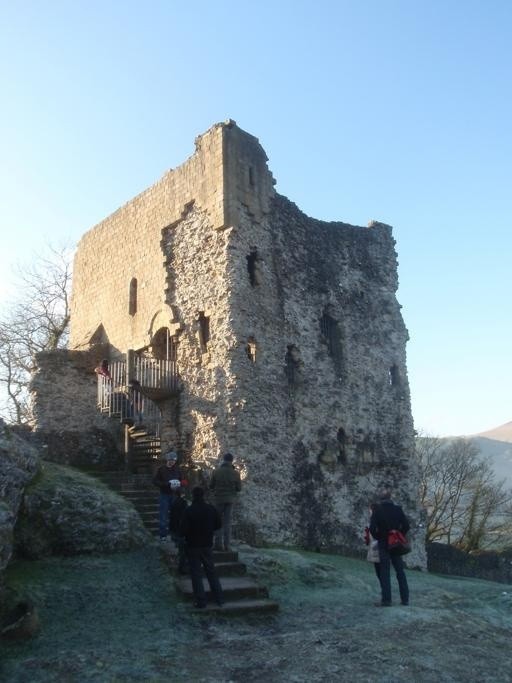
[167,452,178,461]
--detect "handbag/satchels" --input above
[388,529,412,557]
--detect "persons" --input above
[180,486,224,608]
[207,453,241,551]
[97,359,111,379]
[363,503,381,590]
[124,378,142,429]
[155,451,184,543]
[368,488,410,606]
